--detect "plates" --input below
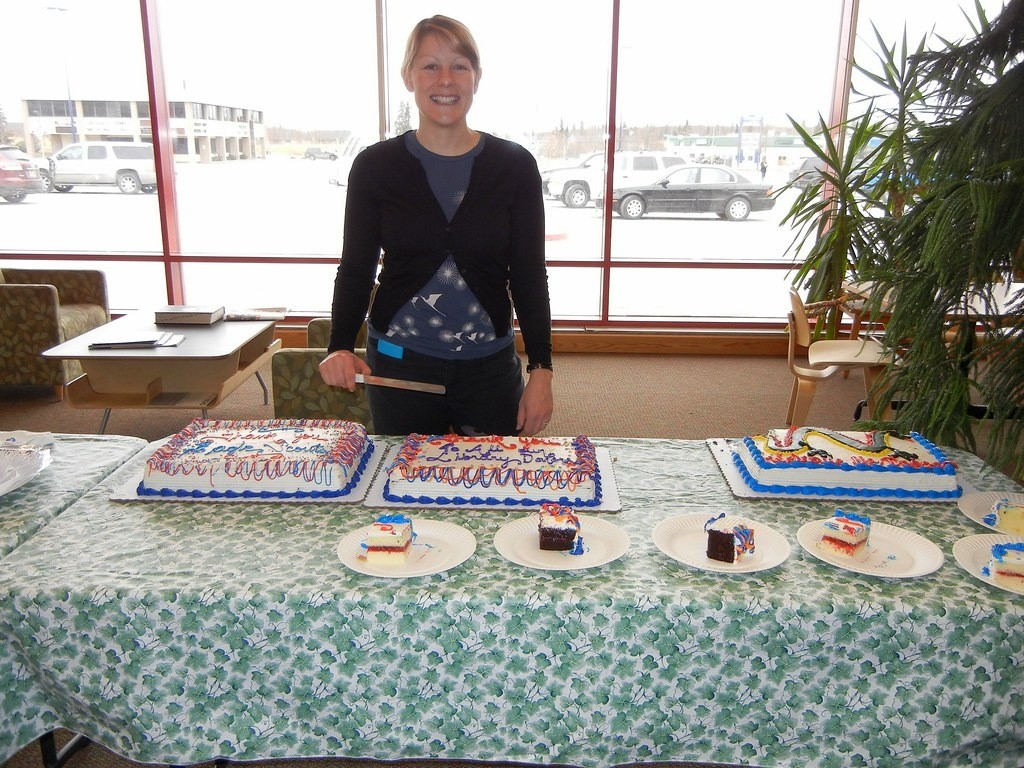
[957,491,1024,536]
[952,533,1024,597]
[493,514,631,571]
[651,512,792,575]
[796,518,945,578]
[337,518,477,579]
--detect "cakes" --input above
[137,418,374,499]
[989,541,1024,593]
[817,509,871,559]
[982,497,1024,537]
[366,513,417,564]
[703,512,755,565]
[537,503,581,552]
[728,424,965,499]
[384,433,602,507]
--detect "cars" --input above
[0,145,41,201]
[305,147,338,161]
[787,156,931,196]
[598,164,775,218]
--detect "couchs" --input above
[0,268,111,401]
[272,317,377,435]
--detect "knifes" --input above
[355,373,447,396]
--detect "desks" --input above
[839,277,1024,428]
[42,304,283,435]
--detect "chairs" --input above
[784,286,894,430]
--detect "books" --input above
[154,305,226,326]
[88,332,185,348]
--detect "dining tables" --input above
[0,432,1024,768]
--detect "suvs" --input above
[547,151,702,208]
[49,139,158,194]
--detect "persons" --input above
[318,16,553,437]
[760,159,768,180]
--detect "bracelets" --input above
[526,364,553,374]
